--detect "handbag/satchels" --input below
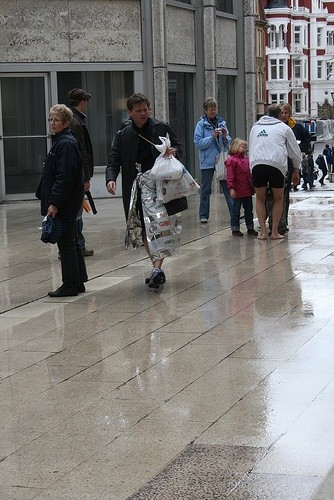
[214,144,231,180]
[149,132,200,204]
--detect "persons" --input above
[224,138,258,236]
[193,98,246,223]
[35,104,89,296]
[331,147,334,173]
[301,153,312,191]
[105,93,182,289]
[323,144,332,172]
[268,102,311,235]
[302,149,316,188]
[315,154,327,186]
[121,102,152,247]
[58,88,94,260]
[289,165,300,191]
[248,104,301,239]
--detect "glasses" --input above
[48,118,62,123]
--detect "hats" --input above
[68,88,92,102]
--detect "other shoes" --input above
[232,230,244,236]
[200,219,208,223]
[145,267,166,290]
[48,283,85,296]
[247,229,259,236]
[83,248,94,256]
